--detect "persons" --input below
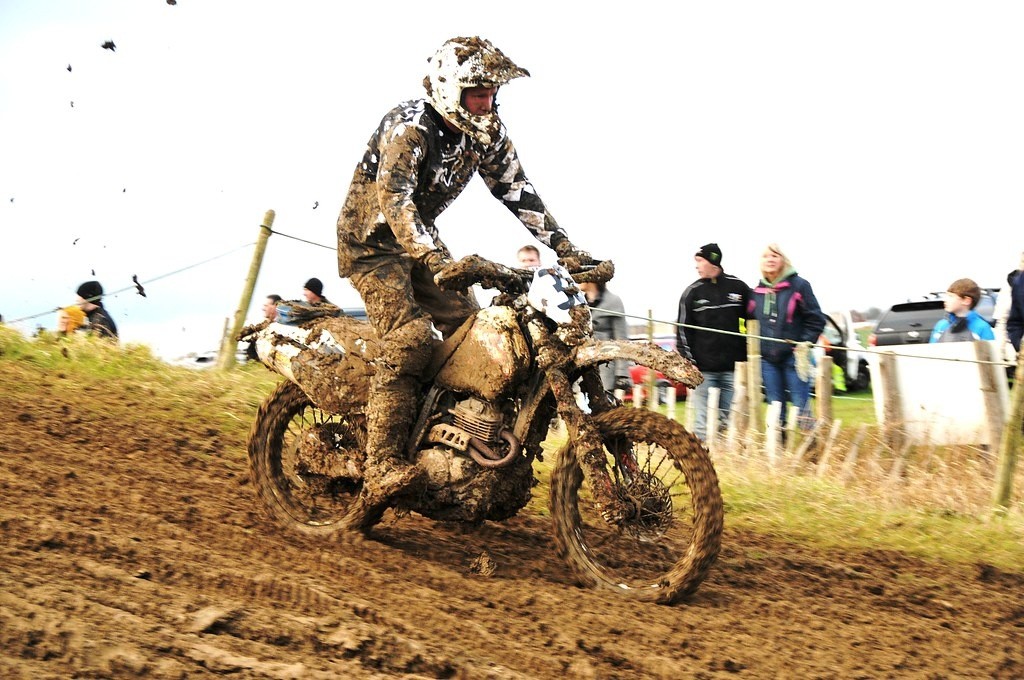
[60,281,117,338]
[518,245,541,271]
[247,294,282,361]
[337,39,606,512]
[748,244,826,447]
[573,259,632,414]
[992,251,1024,365]
[675,244,753,441]
[928,278,994,343]
[304,278,331,305]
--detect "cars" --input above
[182,352,216,370]
[622,338,687,403]
[821,313,869,397]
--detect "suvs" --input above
[870,287,1000,347]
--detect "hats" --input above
[75,281,104,310]
[304,278,323,298]
[695,243,723,267]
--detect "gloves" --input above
[426,250,469,298]
[566,246,594,266]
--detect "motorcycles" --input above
[246,254,723,603]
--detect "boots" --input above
[364,384,430,509]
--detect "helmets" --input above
[422,36,531,152]
